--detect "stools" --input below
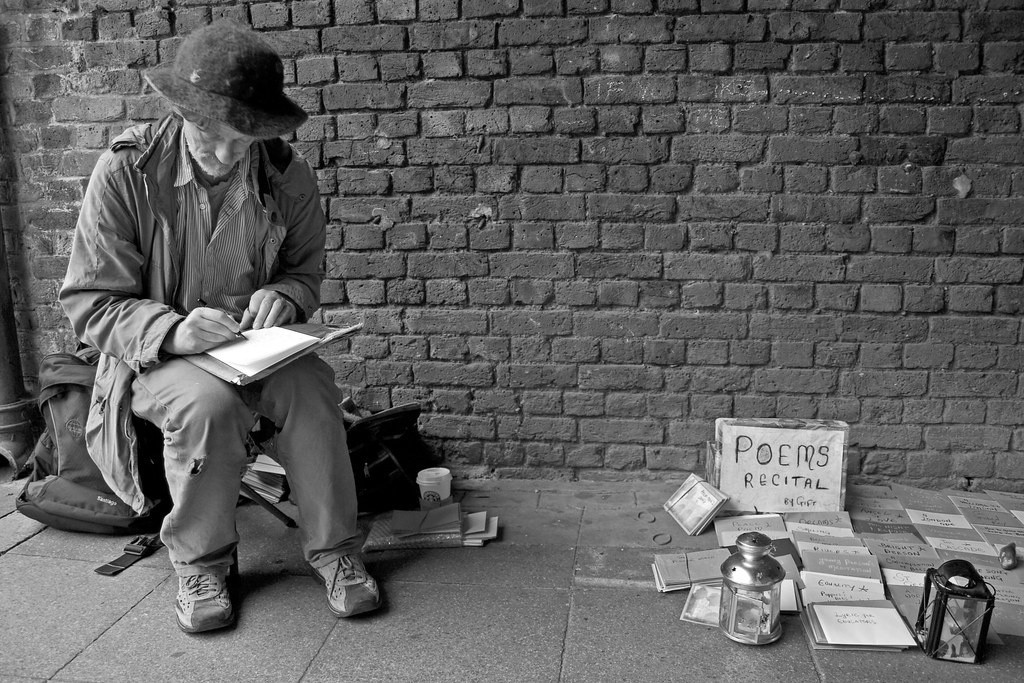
[130,413,299,530]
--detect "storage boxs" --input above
[704,417,850,516]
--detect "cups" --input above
[416,467,453,501]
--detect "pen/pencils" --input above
[196,297,249,340]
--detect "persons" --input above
[58,20,383,634]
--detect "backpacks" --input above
[15,351,173,534]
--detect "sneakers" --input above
[314,552,381,618]
[173,570,234,635]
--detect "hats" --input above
[144,18,311,139]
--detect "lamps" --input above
[913,559,997,665]
[718,532,786,646]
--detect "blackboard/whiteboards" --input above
[713,416,851,518]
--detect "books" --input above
[652,476,1024,651]
[237,455,286,504]
[183,322,361,386]
